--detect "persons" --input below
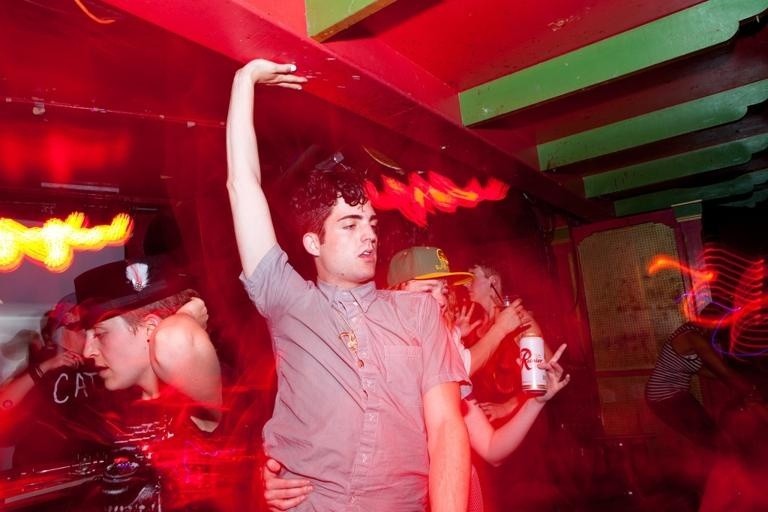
[644,299,758,434]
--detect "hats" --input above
[68,251,193,326]
[387,247,474,292]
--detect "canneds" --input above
[519,333,547,396]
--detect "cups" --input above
[494,296,516,313]
